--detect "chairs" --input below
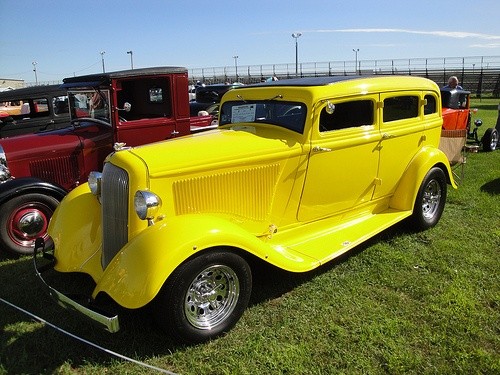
[439,129,467,187]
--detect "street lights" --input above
[126,50,134,69]
[353,48,360,72]
[32,62,38,82]
[100,50,106,73]
[292,32,303,75]
[232,55,239,76]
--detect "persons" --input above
[75,92,105,111]
[441,76,465,109]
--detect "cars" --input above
[440,88,469,153]
[0,83,74,139]
[0,65,219,256]
[188,73,500,153]
[33,75,457,344]
